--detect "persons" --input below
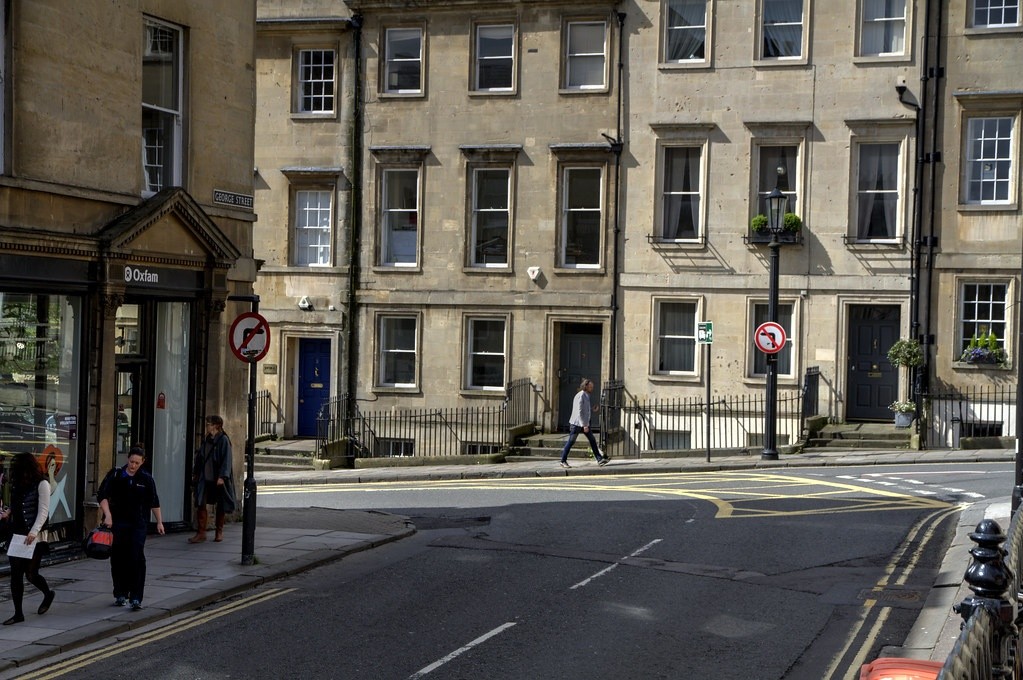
[0,452,56,625]
[188,414,237,543]
[97,444,165,610]
[559,379,611,468]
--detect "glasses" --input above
[206,422,210,425]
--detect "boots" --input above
[215,507,225,541]
[188,510,207,542]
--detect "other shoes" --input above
[598,458,611,467]
[560,461,571,468]
[38,591,55,615]
[3,616,24,625]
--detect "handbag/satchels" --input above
[82,525,113,560]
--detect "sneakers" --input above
[114,596,128,606]
[128,600,142,609]
